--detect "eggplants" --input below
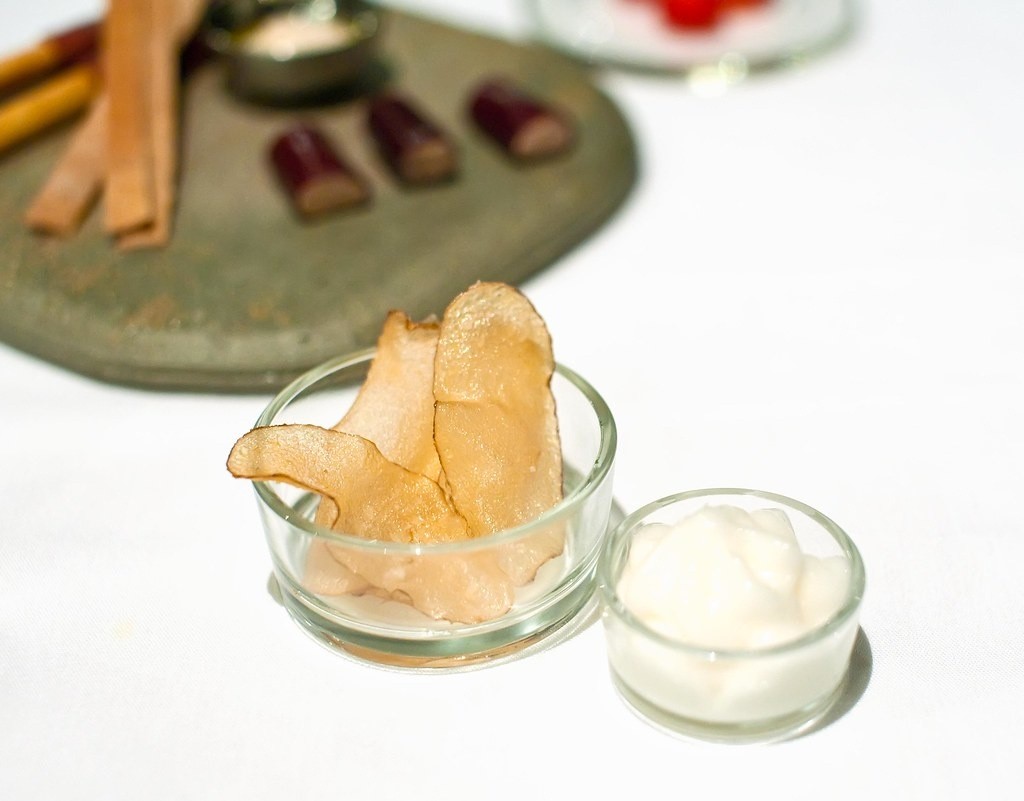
[273,128,364,217]
[472,81,574,163]
[368,96,455,186]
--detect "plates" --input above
[0,0,639,394]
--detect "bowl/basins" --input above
[204,1,387,103]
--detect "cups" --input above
[241,336,618,660]
[609,491,869,741]
